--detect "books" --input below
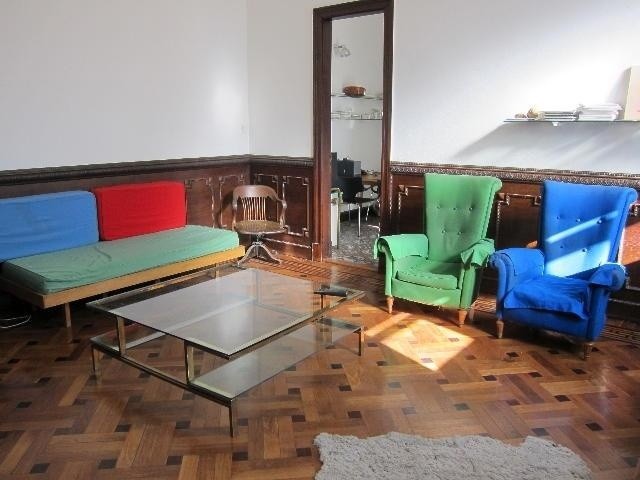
[542,102,623,122]
[624,65,640,121]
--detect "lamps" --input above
[337,42,351,57]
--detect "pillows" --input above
[91,179,187,239]
[0,190,100,264]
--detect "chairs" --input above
[485,179,639,361]
[337,177,379,237]
[374,172,502,328]
[231,184,289,267]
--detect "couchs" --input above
[0,225,246,329]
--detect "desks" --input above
[349,174,381,214]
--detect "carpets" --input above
[312,430,594,480]
